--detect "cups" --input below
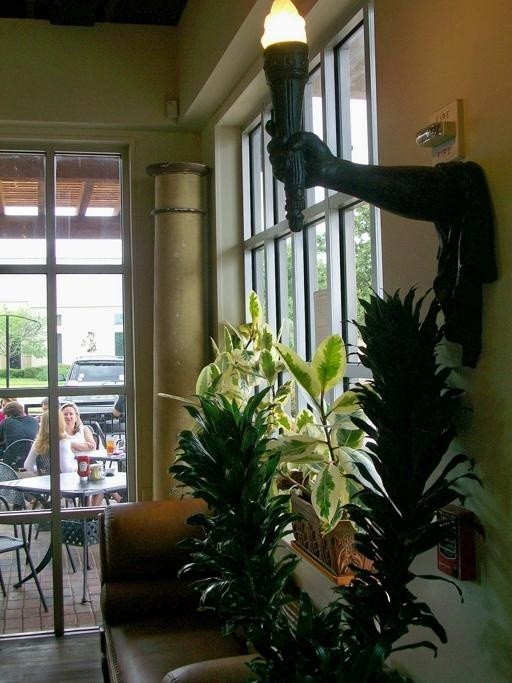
[106,440,114,455]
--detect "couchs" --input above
[98,497,329,683]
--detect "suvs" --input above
[54,356,127,435]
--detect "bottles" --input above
[76,455,91,482]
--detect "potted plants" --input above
[191,288,373,586]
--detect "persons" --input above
[24,409,80,473]
[0,402,41,472]
[261,121,499,366]
[62,401,124,506]
[42,398,61,412]
[0,397,18,412]
[112,393,126,424]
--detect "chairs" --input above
[0,400,125,613]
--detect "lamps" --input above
[261,0,311,231]
